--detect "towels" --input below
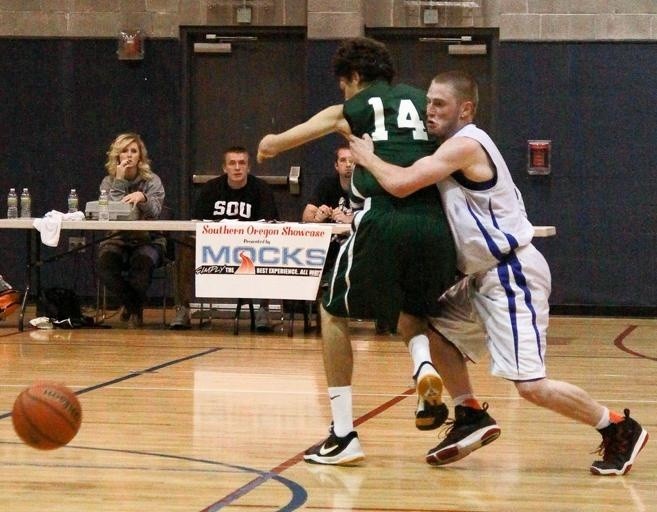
[33,210,85,248]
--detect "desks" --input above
[0,218,557,338]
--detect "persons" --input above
[91,132,166,330]
[169,144,282,332]
[254,36,458,466]
[301,144,364,225]
[347,65,651,479]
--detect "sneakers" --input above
[302,421,365,464]
[254,306,270,331]
[118,284,150,328]
[168,304,192,329]
[425,402,500,465]
[411,362,448,430]
[588,408,649,475]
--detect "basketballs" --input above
[11,383,81,450]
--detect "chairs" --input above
[96,241,180,328]
[199,302,284,332]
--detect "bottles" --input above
[20,188,32,218]
[67,188,79,213]
[7,187,18,218]
[97,189,108,221]
